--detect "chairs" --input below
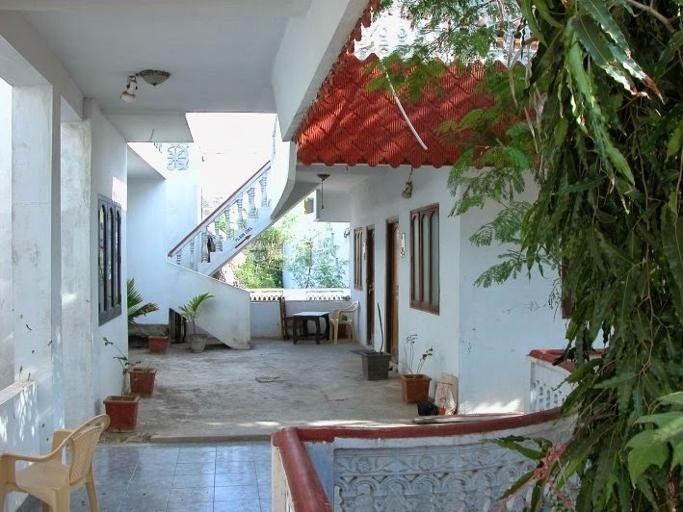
[0,414,111,512]
[329,300,358,344]
[279,296,304,341]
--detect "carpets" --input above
[350,349,370,355]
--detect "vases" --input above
[148,335,168,353]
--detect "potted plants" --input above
[178,292,215,353]
[103,359,140,431]
[399,334,433,404]
[360,304,392,380]
[103,337,157,398]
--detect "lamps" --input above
[400,165,414,198]
[119,73,137,104]
[317,174,330,220]
[140,70,171,87]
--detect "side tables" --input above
[292,312,329,344]
